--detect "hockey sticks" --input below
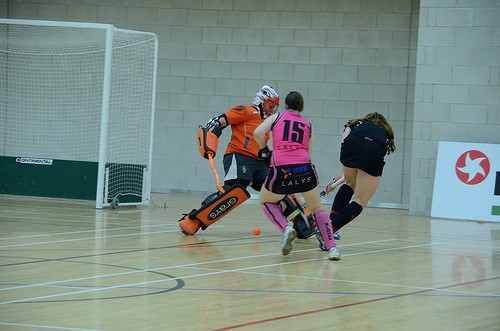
[288,191,342,241]
[206,151,226,193]
[286,177,346,220]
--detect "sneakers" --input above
[315,229,328,250]
[333,232,342,240]
[280,226,297,256]
[328,247,340,260]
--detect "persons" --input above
[315,112,396,251]
[178,85,320,238]
[253,91,340,260]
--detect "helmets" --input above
[252,84,280,118]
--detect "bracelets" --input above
[333,177,336,183]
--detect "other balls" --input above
[253,228,261,236]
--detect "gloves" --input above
[258,145,272,168]
